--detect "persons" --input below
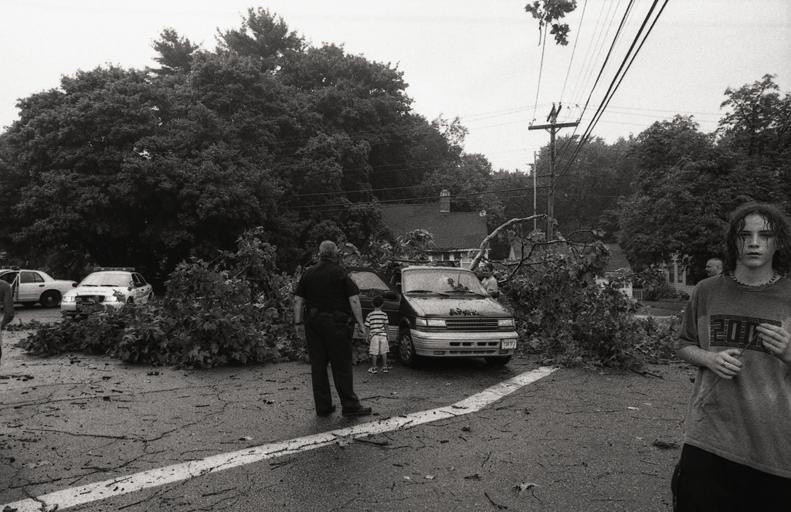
[481,264,498,301]
[293,240,374,417]
[0,279,15,362]
[703,256,724,279]
[363,296,391,373]
[673,201,791,512]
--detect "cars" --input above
[60,265,157,321]
[0,269,78,308]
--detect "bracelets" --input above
[295,321,303,326]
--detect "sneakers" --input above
[383,365,389,373]
[368,367,378,374]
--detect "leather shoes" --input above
[317,404,337,417]
[341,405,371,417]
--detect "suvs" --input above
[341,258,519,369]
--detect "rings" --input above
[721,360,728,366]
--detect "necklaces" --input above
[731,272,780,287]
